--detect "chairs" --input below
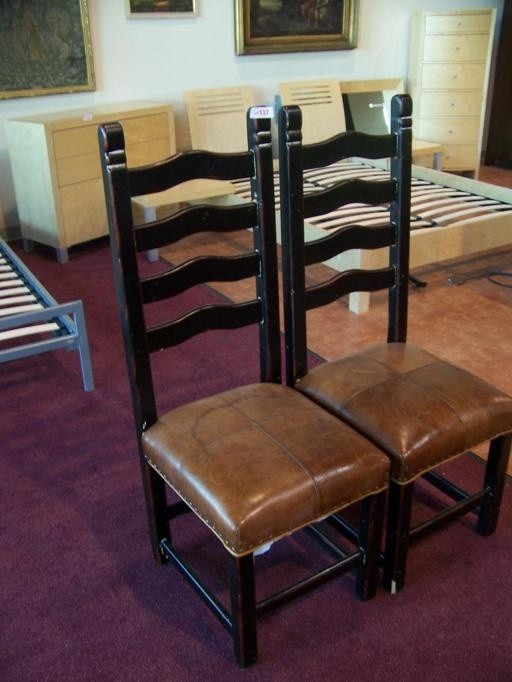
[279,94,511,592]
[102,102,392,668]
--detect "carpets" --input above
[1,236,511,681]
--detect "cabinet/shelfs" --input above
[4,102,182,265]
[406,6,497,181]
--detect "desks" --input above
[411,138,443,172]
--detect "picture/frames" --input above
[3,2,97,98]
[125,0,198,19]
[235,0,357,57]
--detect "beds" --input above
[186,81,511,316]
